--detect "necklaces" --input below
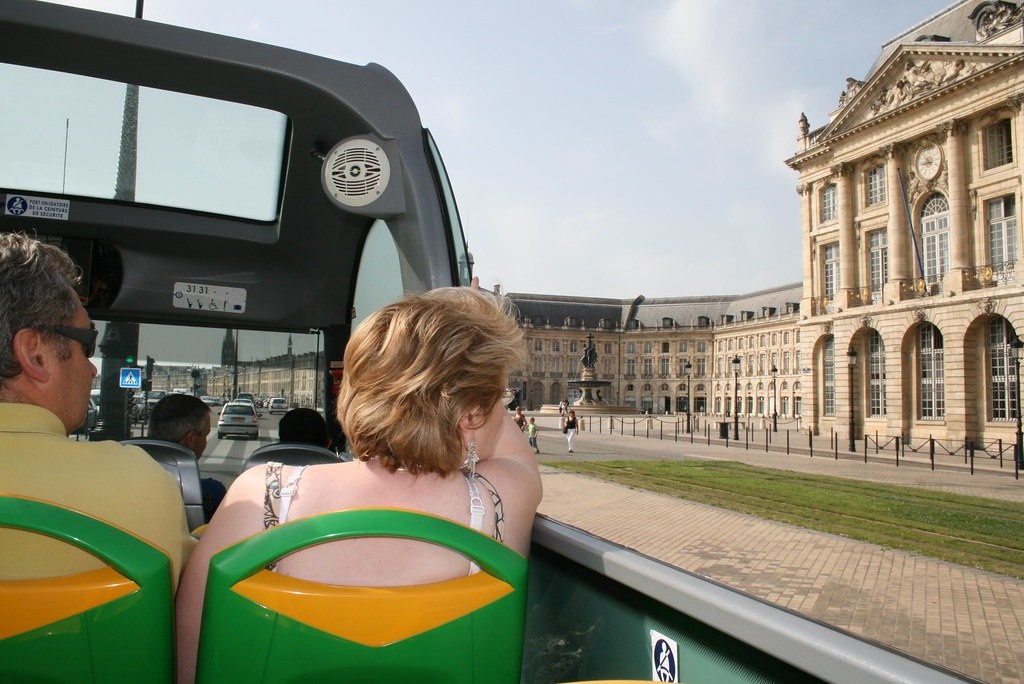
[397,467,408,472]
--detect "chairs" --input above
[242,445,340,472]
[194,507,527,684]
[0,491,175,684]
[119,440,204,531]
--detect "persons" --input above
[559,397,569,415]
[279,408,331,449]
[514,406,527,432]
[173,277,541,684]
[587,344,597,367]
[564,411,579,452]
[580,344,588,367]
[148,394,226,524]
[528,417,540,454]
[871,58,966,116]
[0,233,197,592]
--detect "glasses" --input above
[500,385,515,406]
[31,320,99,358]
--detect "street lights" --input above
[792,391,795,421]
[747,391,752,415]
[1008,335,1024,470]
[771,365,778,434]
[846,346,858,453]
[732,354,741,440]
[685,360,693,436]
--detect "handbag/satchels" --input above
[563,427,567,434]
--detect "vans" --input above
[88,388,101,418]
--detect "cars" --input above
[217,402,263,442]
[267,398,289,414]
[131,386,272,421]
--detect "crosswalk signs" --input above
[120,368,141,389]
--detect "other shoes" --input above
[534,449,539,453]
[569,449,573,452]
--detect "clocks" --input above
[913,134,946,182]
